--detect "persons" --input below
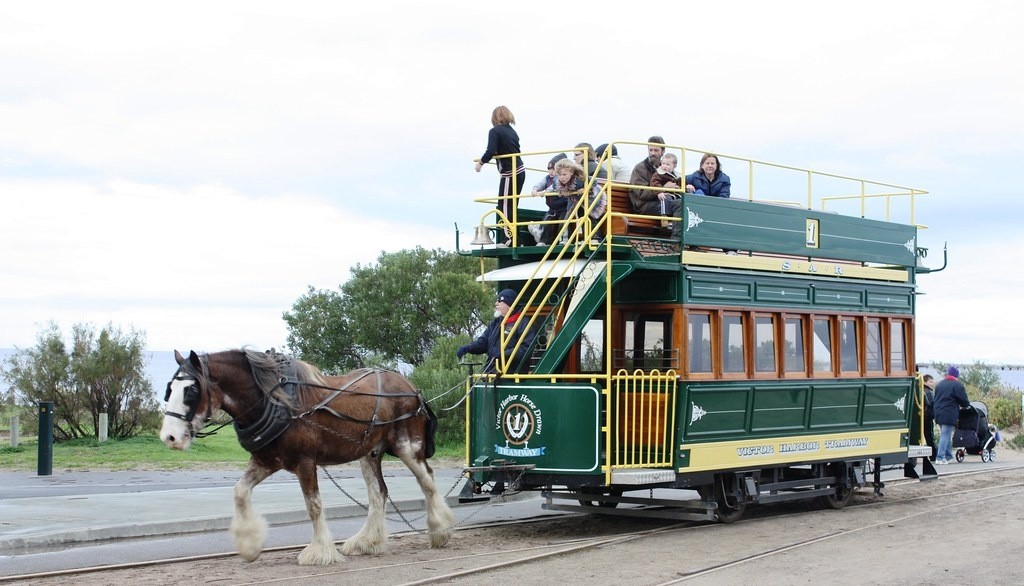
[475,105,524,248]
[528,136,740,256]
[933,367,970,464]
[923,374,938,461]
[457,289,536,382]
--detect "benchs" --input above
[598,181,662,236]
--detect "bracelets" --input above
[479,161,484,165]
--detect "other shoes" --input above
[669,240,739,257]
[495,221,600,248]
[661,215,669,227]
[930,456,936,464]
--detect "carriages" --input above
[152,138,946,566]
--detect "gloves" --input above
[491,352,501,361]
[457,346,469,358]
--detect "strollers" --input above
[954,401,1002,462]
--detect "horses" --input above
[159,345,454,566]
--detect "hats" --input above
[947,366,960,378]
[595,144,618,157]
[497,289,519,309]
[548,152,567,169]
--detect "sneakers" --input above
[935,459,948,465]
[946,458,958,463]
